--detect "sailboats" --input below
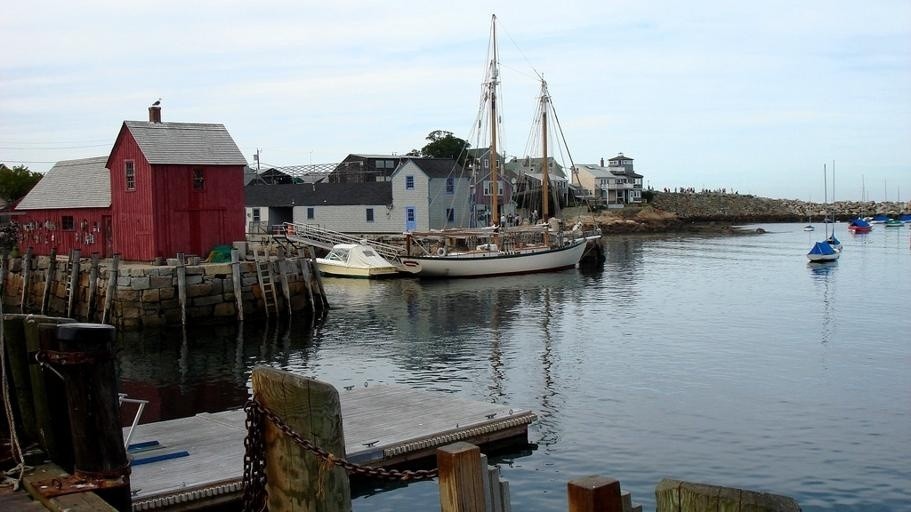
[393,12,602,281]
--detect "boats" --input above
[847,174,910,234]
[804,202,814,231]
[309,239,399,279]
[806,163,839,262]
[826,160,842,251]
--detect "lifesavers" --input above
[437,248,446,256]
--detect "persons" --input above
[501,209,539,226]
